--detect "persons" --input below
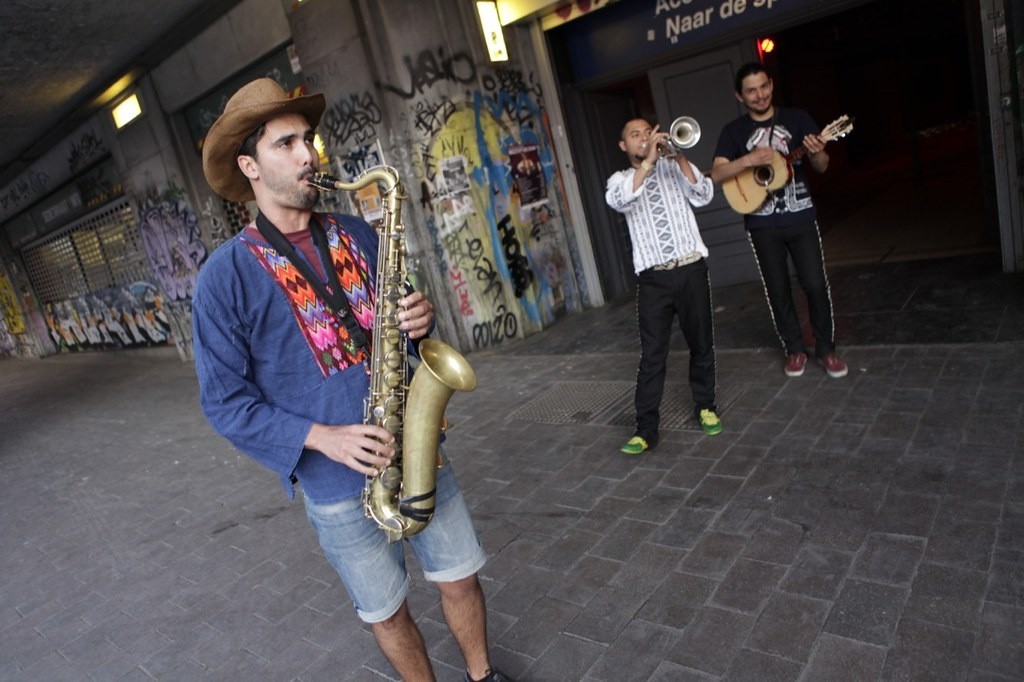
[191,78,512,682]
[711,63,850,379]
[605,114,724,454]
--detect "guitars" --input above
[722,114,853,214]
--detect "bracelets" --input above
[641,159,655,174]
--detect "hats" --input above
[200,78,327,202]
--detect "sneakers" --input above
[818,351,848,377]
[699,408,723,435]
[464,667,517,682]
[619,435,648,454]
[784,351,808,376]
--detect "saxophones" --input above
[306,164,478,543]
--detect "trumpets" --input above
[641,116,701,158]
